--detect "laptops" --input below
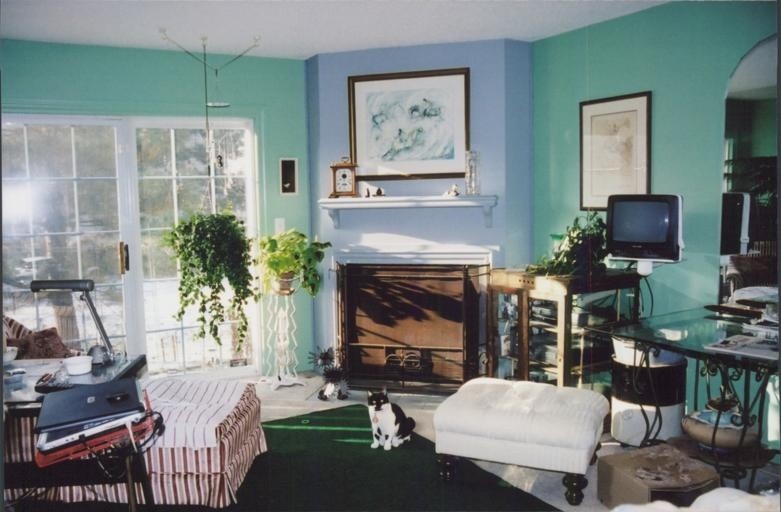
[35,376,146,453]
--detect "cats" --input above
[367,384,416,451]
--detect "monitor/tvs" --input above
[721,192,751,268]
[606,193,682,263]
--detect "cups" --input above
[64,356,93,376]
[465,151,482,196]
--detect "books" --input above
[704,332,781,363]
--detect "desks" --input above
[1,354,151,509]
[486,268,644,387]
[582,307,781,492]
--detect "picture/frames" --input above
[579,90,654,213]
[347,66,472,182]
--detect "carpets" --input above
[231,402,568,511]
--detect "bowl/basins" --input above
[2,347,18,363]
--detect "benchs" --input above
[2,316,267,508]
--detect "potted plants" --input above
[252,230,332,299]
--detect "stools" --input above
[431,375,611,508]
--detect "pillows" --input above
[5,325,73,360]
